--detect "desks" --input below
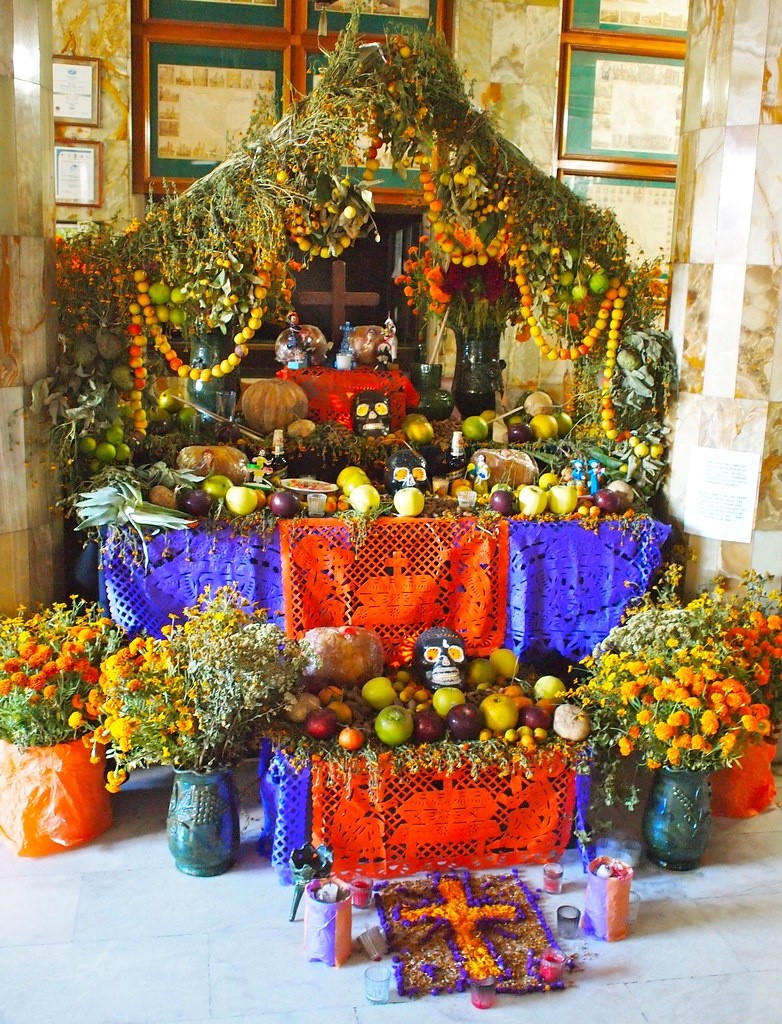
[256,719,600,877]
[97,509,668,668]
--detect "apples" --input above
[80,117,665,517]
[301,646,565,740]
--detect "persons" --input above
[353,391,390,438]
[471,453,491,497]
[251,448,272,482]
[585,460,606,496]
[288,313,301,362]
[415,626,470,692]
[381,449,429,493]
[567,458,586,485]
[383,318,398,360]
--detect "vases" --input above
[0,735,114,857]
[450,325,503,419]
[187,330,228,413]
[712,734,777,819]
[407,361,455,422]
[164,763,240,877]
[647,763,715,871]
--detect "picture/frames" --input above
[51,54,104,130]
[53,138,103,209]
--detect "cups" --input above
[557,905,579,938]
[217,391,236,421]
[628,891,640,924]
[364,965,391,1004]
[307,493,327,517]
[356,925,385,960]
[458,491,477,512]
[337,353,352,370]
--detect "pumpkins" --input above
[344,325,390,365]
[553,704,592,741]
[177,446,250,483]
[242,378,309,434]
[470,447,537,487]
[286,691,322,722]
[274,323,327,364]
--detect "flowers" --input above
[1,581,309,795]
[424,259,525,339]
[559,576,782,810]
[183,255,265,337]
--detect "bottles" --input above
[266,429,289,487]
[446,431,468,495]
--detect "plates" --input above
[281,480,338,496]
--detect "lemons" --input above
[490,648,520,679]
